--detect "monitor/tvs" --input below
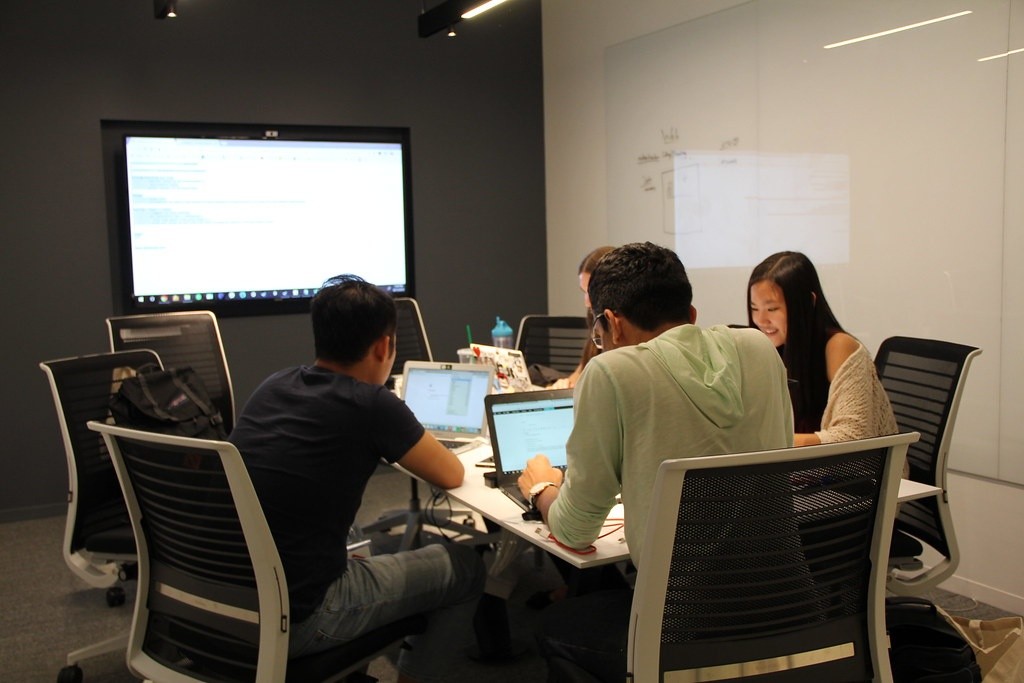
[97,117,417,318]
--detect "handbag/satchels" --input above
[934,605,1024,683]
[857,593,983,683]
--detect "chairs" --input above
[627,431,923,683]
[379,296,434,392]
[514,315,590,374]
[106,309,236,436]
[56,420,429,683]
[887,335,982,593]
[41,347,174,588]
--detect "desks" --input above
[348,443,940,572]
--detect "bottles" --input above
[491,316,514,349]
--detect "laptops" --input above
[401,361,494,454]
[484,387,574,511]
[469,343,535,392]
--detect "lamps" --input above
[417,0,508,39]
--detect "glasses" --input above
[591,309,616,349]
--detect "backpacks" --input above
[108,365,226,469]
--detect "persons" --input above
[516,240,825,683]
[528,246,643,609]
[168,276,487,683]
[746,251,910,486]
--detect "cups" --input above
[391,374,403,399]
[457,348,470,363]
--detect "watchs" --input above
[528,481,558,507]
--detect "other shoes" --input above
[525,584,586,610]
